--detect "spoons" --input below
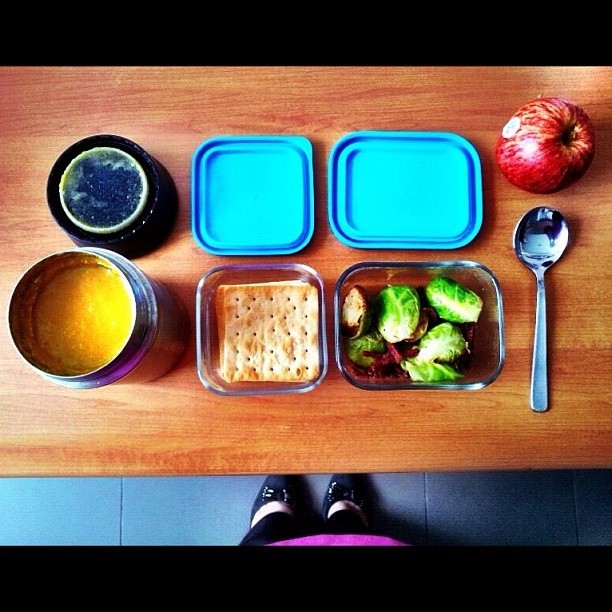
[512,204,570,412]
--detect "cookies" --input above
[216,279,320,384]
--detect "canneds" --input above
[44,133,179,262]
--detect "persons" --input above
[227,474,417,552]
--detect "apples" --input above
[494,97,596,195]
